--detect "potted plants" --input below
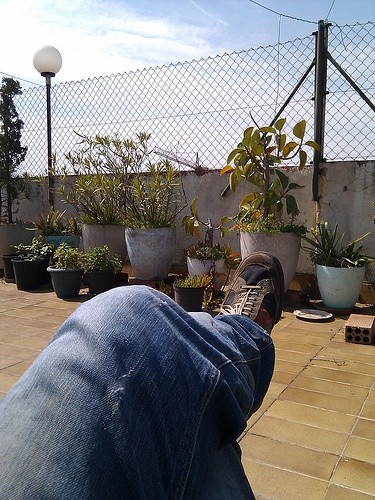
[0,77,374,312]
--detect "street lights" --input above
[33,44,62,202]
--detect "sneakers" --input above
[219,251,285,335]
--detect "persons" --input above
[0,252,286,499]
